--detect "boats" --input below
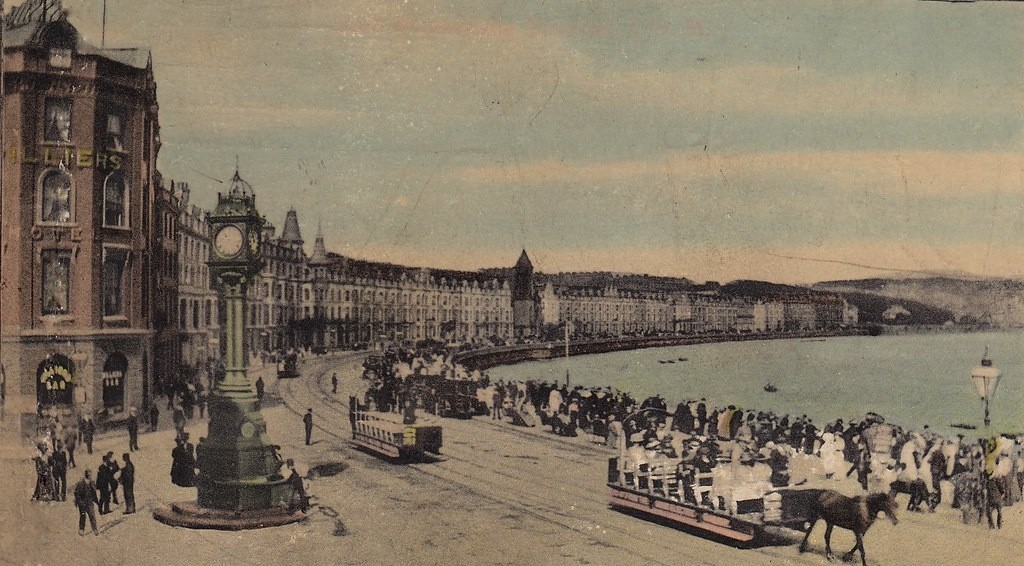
[764,382,776,392]
[658,356,688,363]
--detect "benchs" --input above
[613,452,826,523]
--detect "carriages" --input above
[347,394,444,461]
[606,407,900,566]
[276,350,305,379]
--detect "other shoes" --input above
[122,511,132,514]
[94,530,98,535]
[492,417,497,420]
[130,449,133,452]
[498,416,502,420]
[113,499,119,505]
[73,463,76,468]
[100,511,103,515]
[78,530,84,536]
[107,510,113,513]
[62,496,67,502]
[134,446,138,450]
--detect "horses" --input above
[791,487,900,566]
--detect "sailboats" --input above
[981,345,993,366]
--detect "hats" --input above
[753,451,771,460]
[835,418,844,424]
[629,420,637,427]
[833,431,843,435]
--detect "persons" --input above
[32,357,226,536]
[332,373,337,393]
[364,325,1024,529]
[303,408,313,445]
[251,346,329,363]
[256,376,264,398]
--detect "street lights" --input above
[970,342,1003,441]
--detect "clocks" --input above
[213,224,246,259]
